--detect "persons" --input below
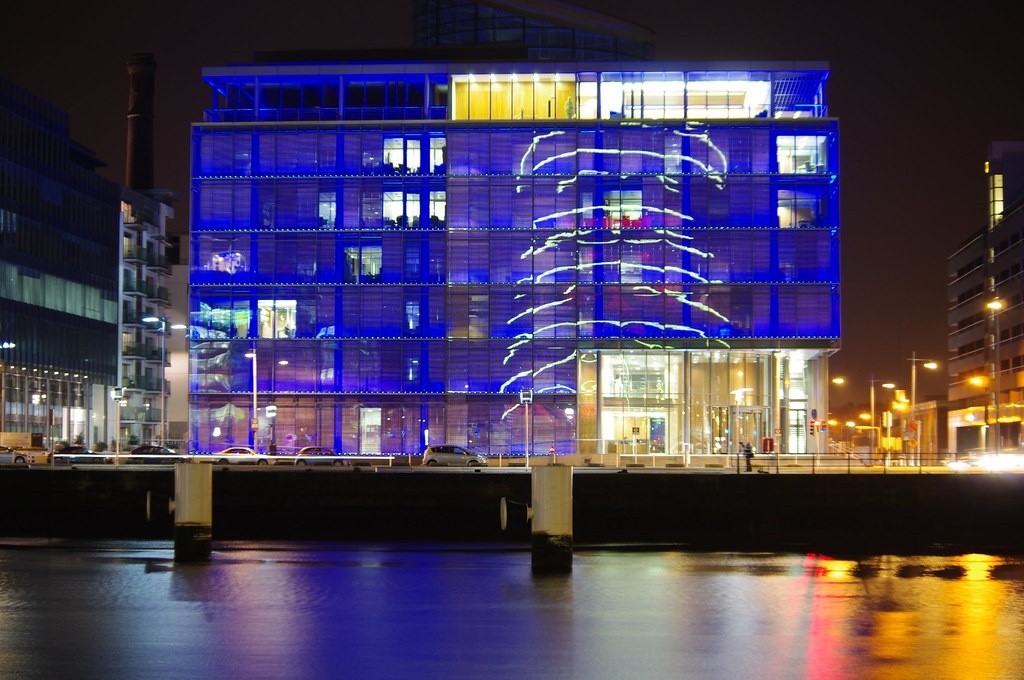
[564,96,574,119]
[742,441,756,472]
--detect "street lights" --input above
[245,352,259,453]
[987,297,1002,455]
[111,388,123,465]
[730,387,753,454]
[142,316,166,446]
[907,351,937,405]
[520,388,533,471]
[831,373,895,464]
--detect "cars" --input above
[422,444,489,468]
[212,447,276,466]
[128,445,192,465]
[47,446,112,464]
[0,446,35,464]
[294,445,352,466]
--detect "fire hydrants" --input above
[743,443,754,471]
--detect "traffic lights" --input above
[809,422,814,436]
[821,420,827,433]
